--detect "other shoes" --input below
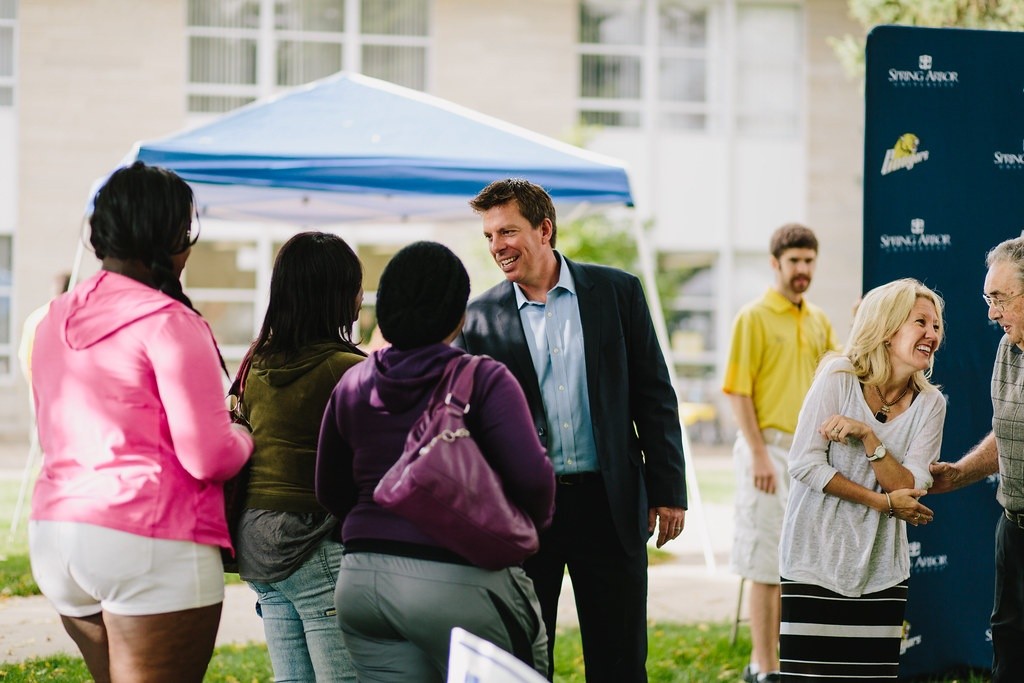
[745,665,780,683]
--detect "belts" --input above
[557,473,601,489]
[1005,508,1024,529]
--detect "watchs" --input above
[866,443,887,462]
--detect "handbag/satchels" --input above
[373,354,539,570]
[221,354,255,573]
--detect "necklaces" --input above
[873,384,906,423]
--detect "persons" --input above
[723,224,844,683]
[453,181,688,683]
[28,160,254,683]
[780,278,944,683]
[927,237,1024,683]
[235,232,558,683]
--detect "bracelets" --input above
[884,491,893,518]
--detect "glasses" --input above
[983,293,1024,311]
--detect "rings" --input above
[915,514,921,519]
[833,428,840,433]
[675,527,680,530]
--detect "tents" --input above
[66,71,716,571]
